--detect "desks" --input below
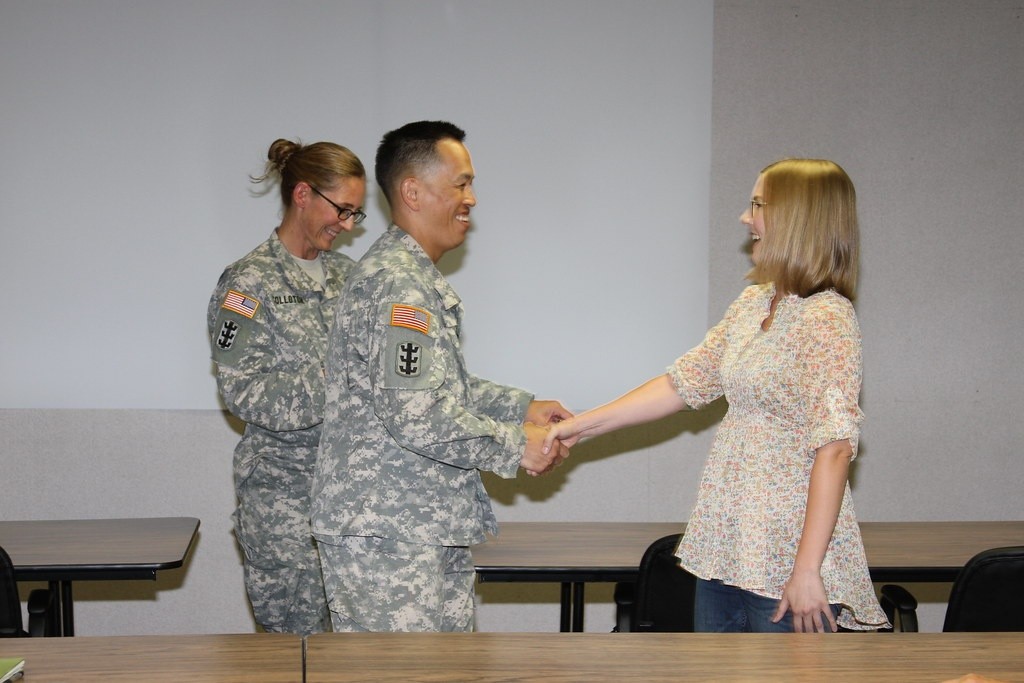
[304,629,1024,683]
[471,519,1024,632]
[0,634,303,683]
[0,516,201,638]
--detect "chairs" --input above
[614,533,1023,633]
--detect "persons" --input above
[308,120,575,634]
[207,139,369,633]
[516,159,894,633]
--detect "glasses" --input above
[307,184,366,225]
[749,199,764,219]
